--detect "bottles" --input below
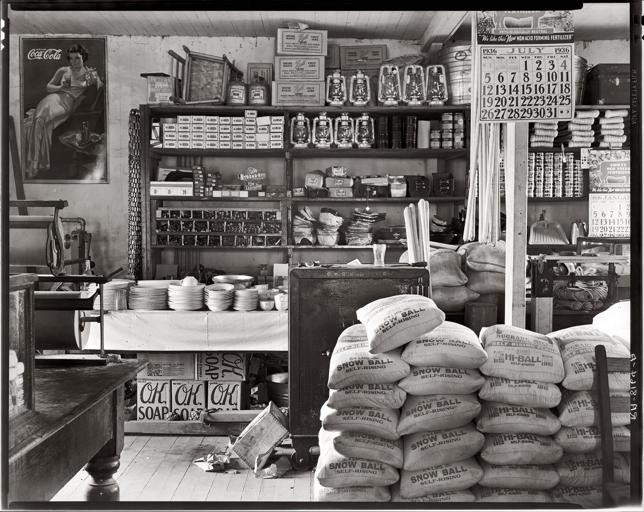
[389,175,408,198]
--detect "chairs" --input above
[593,341,630,507]
[165,45,244,103]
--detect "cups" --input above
[372,243,386,266]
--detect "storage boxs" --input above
[271,25,387,106]
[585,60,629,99]
[146,76,176,104]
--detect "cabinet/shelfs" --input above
[83,104,629,437]
[285,264,432,473]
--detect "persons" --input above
[20,43,104,179]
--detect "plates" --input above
[92,279,259,312]
[353,207,386,223]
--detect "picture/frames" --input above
[18,34,109,185]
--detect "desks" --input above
[8,359,151,504]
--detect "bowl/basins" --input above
[181,276,198,286]
[430,215,447,234]
[212,274,255,291]
[291,205,344,246]
[266,372,289,407]
[253,283,288,311]
